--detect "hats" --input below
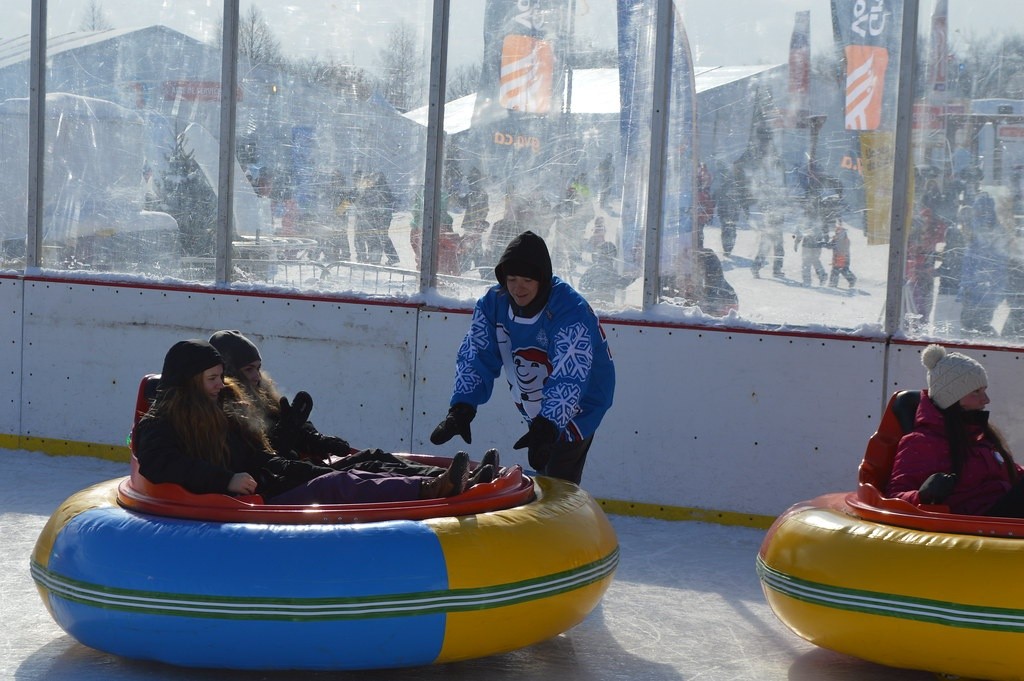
[209,330,262,365]
[921,344,988,410]
[161,339,223,378]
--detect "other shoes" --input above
[467,465,493,486]
[473,448,500,475]
[419,451,469,497]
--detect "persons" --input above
[890,343,1023,517]
[132,327,498,503]
[248,149,1021,339]
[430,230,618,489]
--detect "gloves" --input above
[313,432,350,456]
[919,472,953,503]
[513,417,558,471]
[430,404,477,445]
[278,391,312,435]
